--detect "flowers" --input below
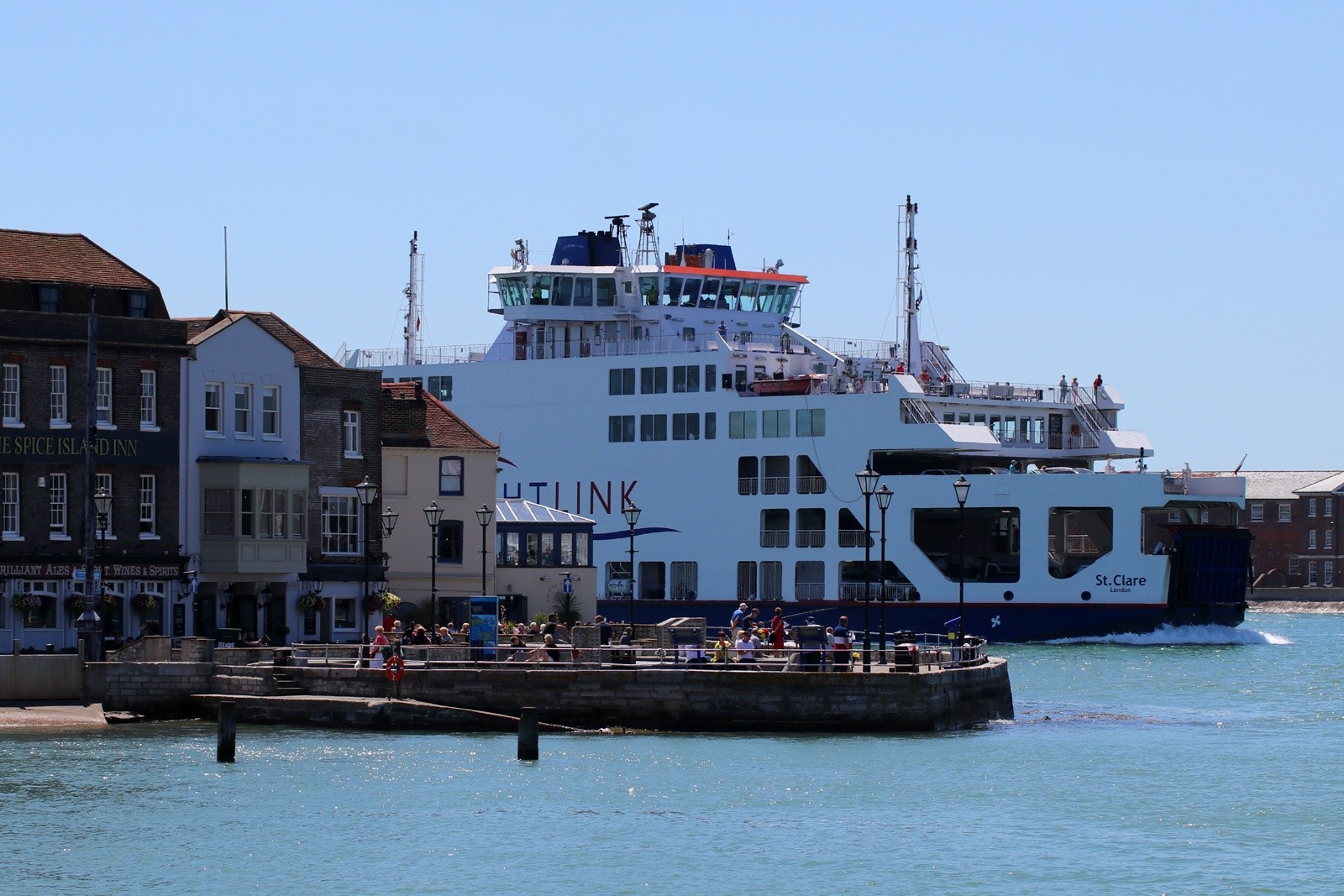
[64,593,119,630]
[131,594,158,613]
[9,593,42,610]
[363,591,402,618]
[297,591,325,617]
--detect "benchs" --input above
[455,352,487,363]
[790,345,817,356]
[745,342,780,353]
[820,380,1037,402]
[708,341,743,352]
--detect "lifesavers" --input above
[386,655,404,681]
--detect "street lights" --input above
[954,474,972,646]
[355,475,380,668]
[855,457,880,671]
[423,501,445,643]
[874,483,894,666]
[381,506,400,539]
[475,503,495,596]
[94,486,114,662]
[622,500,642,657]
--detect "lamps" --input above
[258,582,274,611]
[177,574,192,601]
[0,572,6,596]
[376,507,399,541]
[221,582,239,611]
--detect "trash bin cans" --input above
[894,643,920,672]
[217,628,243,648]
[273,647,296,665]
[611,644,637,670]
[959,635,980,667]
[893,630,916,645]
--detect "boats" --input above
[330,195,1254,644]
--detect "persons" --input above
[1093,374,1102,404]
[1009,460,1018,473]
[781,331,790,353]
[239,632,258,647]
[353,601,872,665]
[1151,534,1169,555]
[835,360,1012,400]
[1059,374,1068,403]
[111,636,122,650]
[1070,377,1079,404]
[1040,465,1047,474]
[646,285,658,305]
[533,282,542,305]
[247,631,259,647]
[718,320,727,342]
